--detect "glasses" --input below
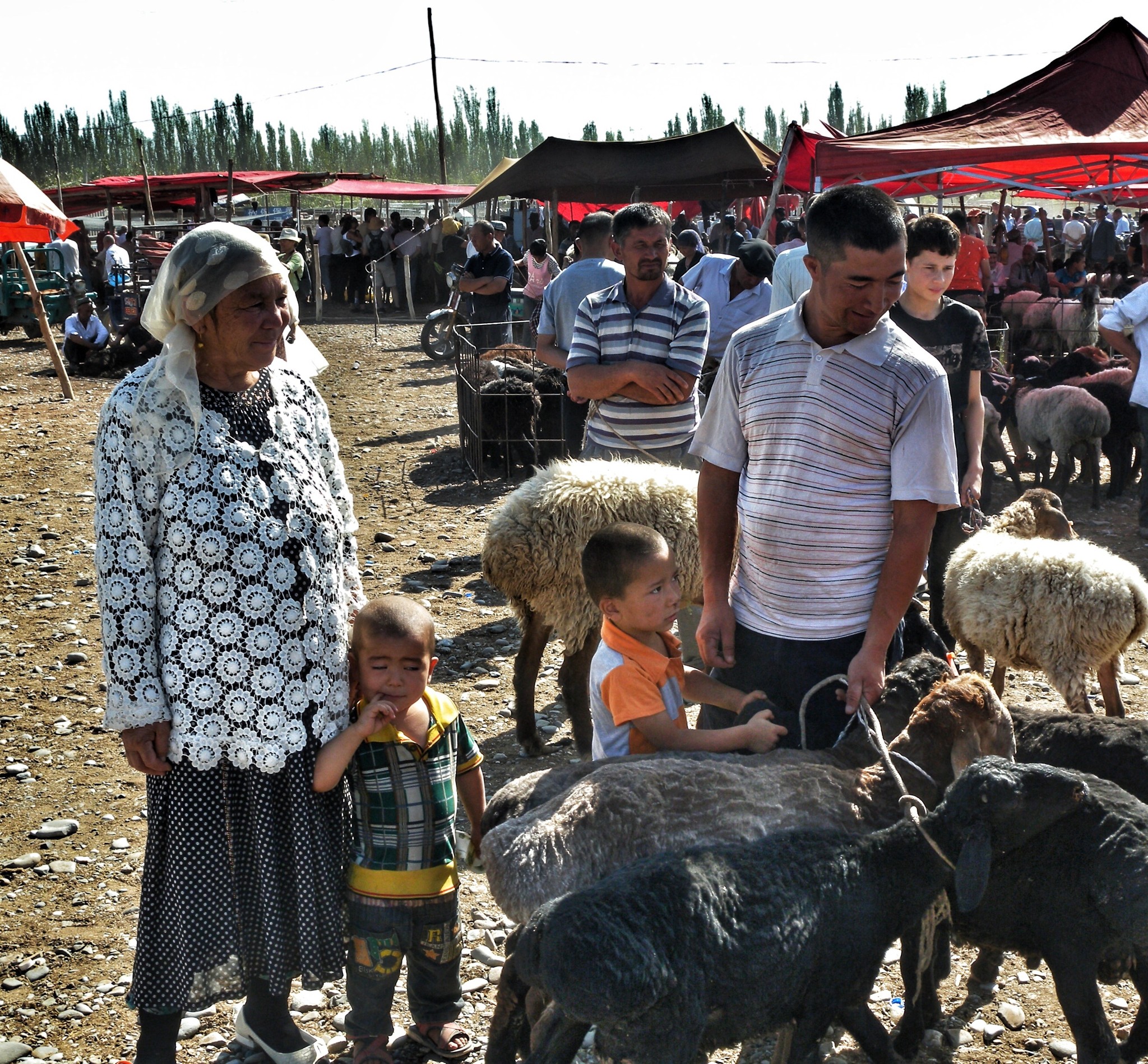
[974,215,983,219]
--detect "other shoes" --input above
[1139,526,1148,539]
[326,294,470,314]
[68,362,80,374]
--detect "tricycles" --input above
[420,263,526,361]
[0,248,98,338]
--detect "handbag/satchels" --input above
[339,235,363,257]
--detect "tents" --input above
[452,122,809,267]
[291,176,540,230]
[40,162,387,238]
[754,16,1148,240]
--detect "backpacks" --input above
[367,231,386,262]
[287,253,312,302]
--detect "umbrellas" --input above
[0,156,81,245]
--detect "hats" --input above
[273,228,302,243]
[528,212,540,219]
[1006,228,1021,239]
[738,238,777,278]
[1063,209,1071,213]
[1078,211,1087,217]
[491,219,507,231]
[725,214,735,224]
[1128,213,1132,217]
[905,213,919,229]
[368,217,384,230]
[1073,212,1080,217]
[991,200,1000,205]
[967,207,986,223]
[1092,205,1108,211]
[986,245,997,254]
[76,297,93,306]
[1026,242,1038,251]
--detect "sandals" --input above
[345,1031,393,1064]
[407,1019,474,1058]
[233,1004,329,1064]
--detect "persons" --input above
[95,221,372,1064]
[247,217,305,296]
[671,237,778,412]
[511,237,564,353]
[1093,281,1148,529]
[31,219,139,298]
[309,204,761,315]
[47,230,82,333]
[109,294,163,359]
[458,220,515,356]
[885,211,994,657]
[667,182,962,754]
[312,596,489,1064]
[770,202,1148,312]
[578,523,788,760]
[556,202,707,472]
[62,297,109,371]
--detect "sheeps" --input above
[472,644,1146,1064]
[458,341,592,479]
[976,281,1147,511]
[479,450,740,759]
[941,486,1148,719]
